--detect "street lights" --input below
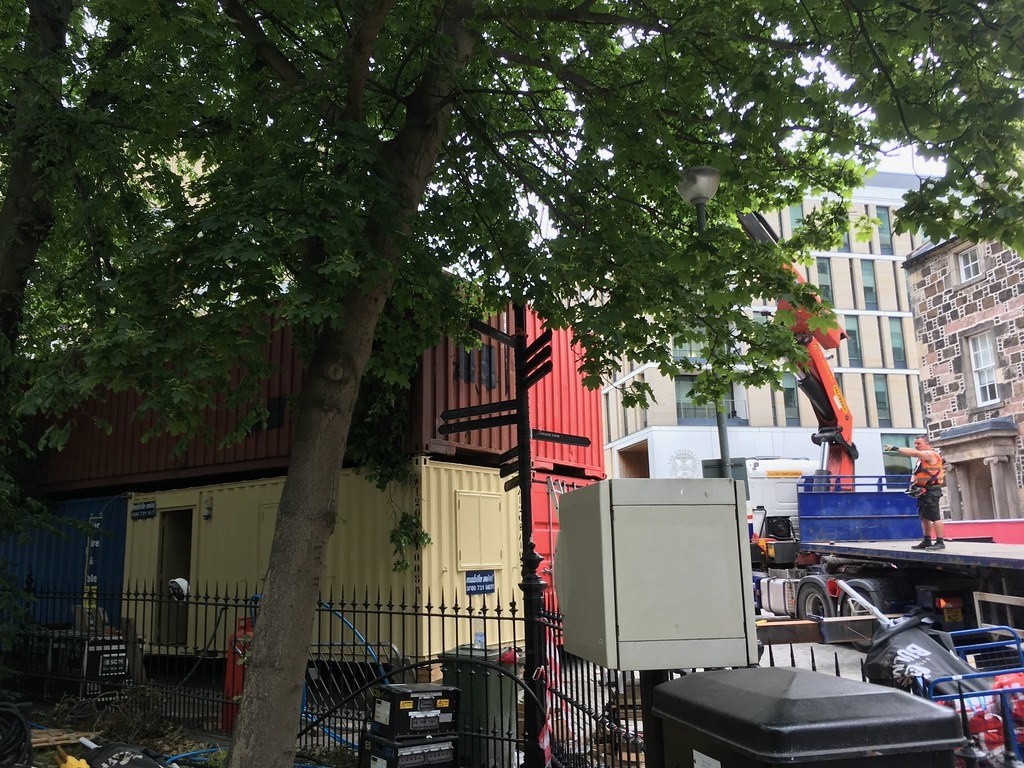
[674,165,732,474]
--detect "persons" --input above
[884,435,945,551]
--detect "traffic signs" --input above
[497,444,521,493]
[469,317,516,351]
[521,315,554,390]
[437,398,522,436]
[531,429,590,448]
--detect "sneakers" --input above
[912,541,931,550]
[925,542,946,551]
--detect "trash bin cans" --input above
[437,643,525,768]
[650,666,967,768]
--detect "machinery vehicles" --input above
[732,194,1024,655]
